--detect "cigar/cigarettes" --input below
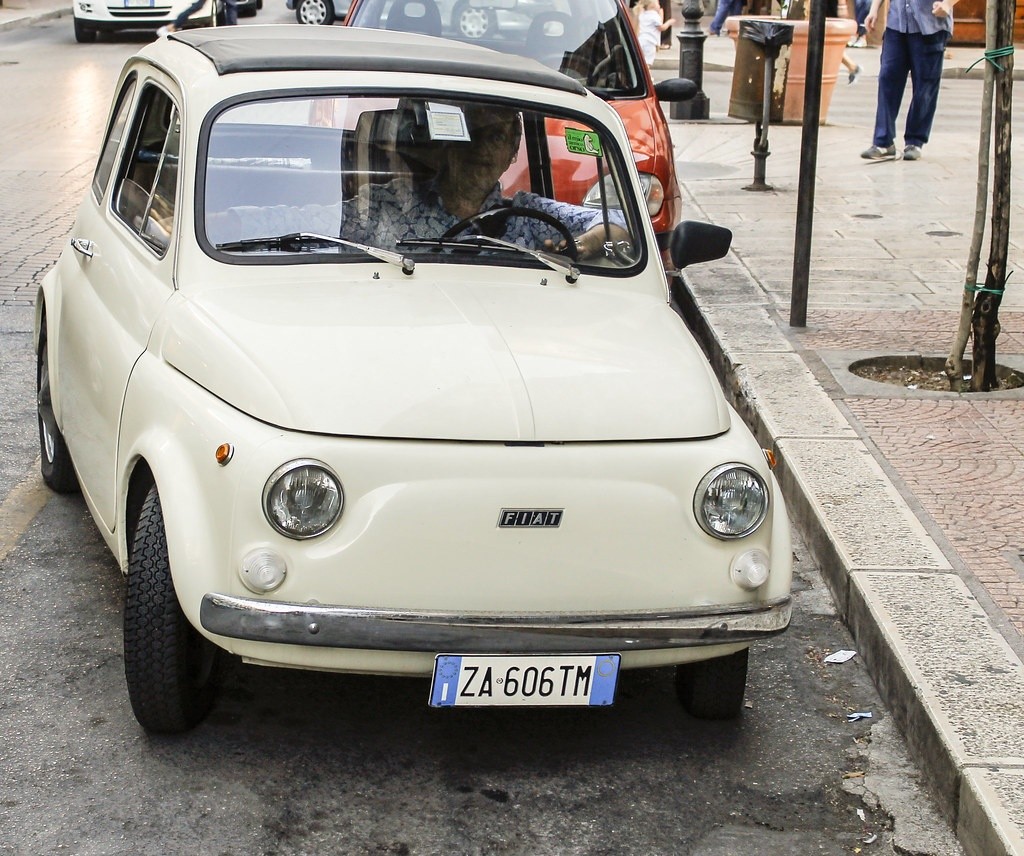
[860,24,865,26]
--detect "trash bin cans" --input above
[727,20,795,123]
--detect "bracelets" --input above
[836,4,847,9]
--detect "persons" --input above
[860,0,960,160]
[637,0,676,67]
[156,0,238,36]
[154,105,635,258]
[847,0,874,47]
[705,0,743,36]
[826,0,861,86]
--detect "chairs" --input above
[353,108,417,196]
[125,93,178,221]
[524,11,576,59]
[385,0,442,37]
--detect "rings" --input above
[574,239,580,246]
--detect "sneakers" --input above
[903,144,922,160]
[860,144,896,162]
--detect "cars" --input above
[286,0,571,40]
[310,0,697,259]
[74,1,230,42]
[36,25,795,738]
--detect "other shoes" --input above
[157,24,183,37]
[848,65,862,86]
